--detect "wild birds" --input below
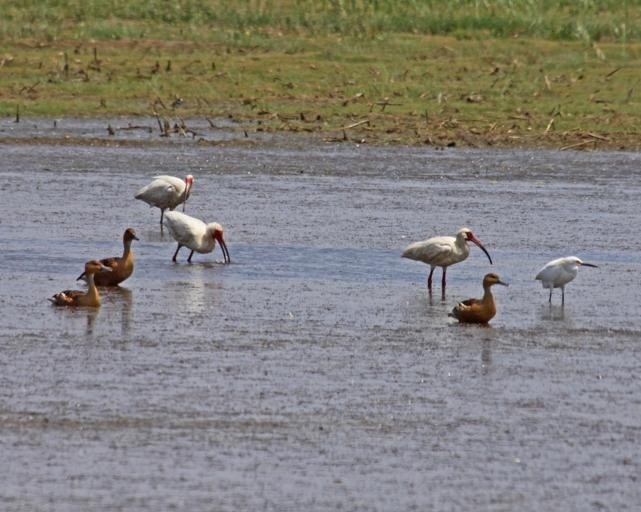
[162,211,230,263]
[448,273,509,323]
[75,227,139,287]
[535,255,599,304]
[401,227,493,299]
[134,174,194,223]
[47,259,113,308]
